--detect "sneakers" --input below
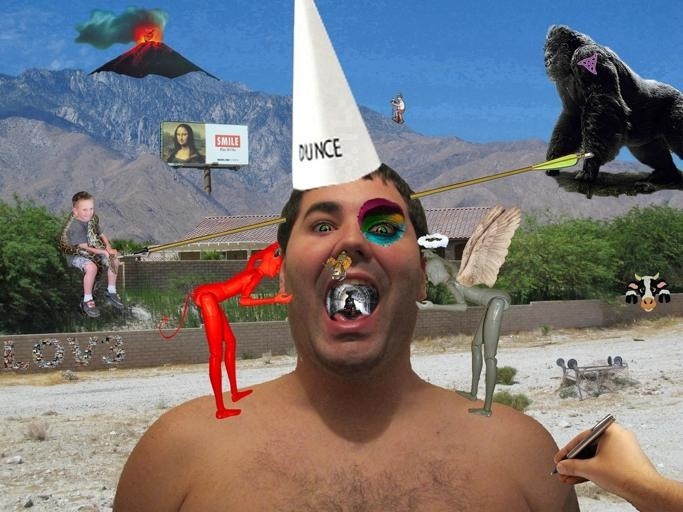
[102,288,125,310]
[78,294,101,318]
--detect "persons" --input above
[165,125,204,165]
[553,414,683,512]
[192,241,294,418]
[112,165,579,512]
[415,252,511,417]
[60,192,127,320]
